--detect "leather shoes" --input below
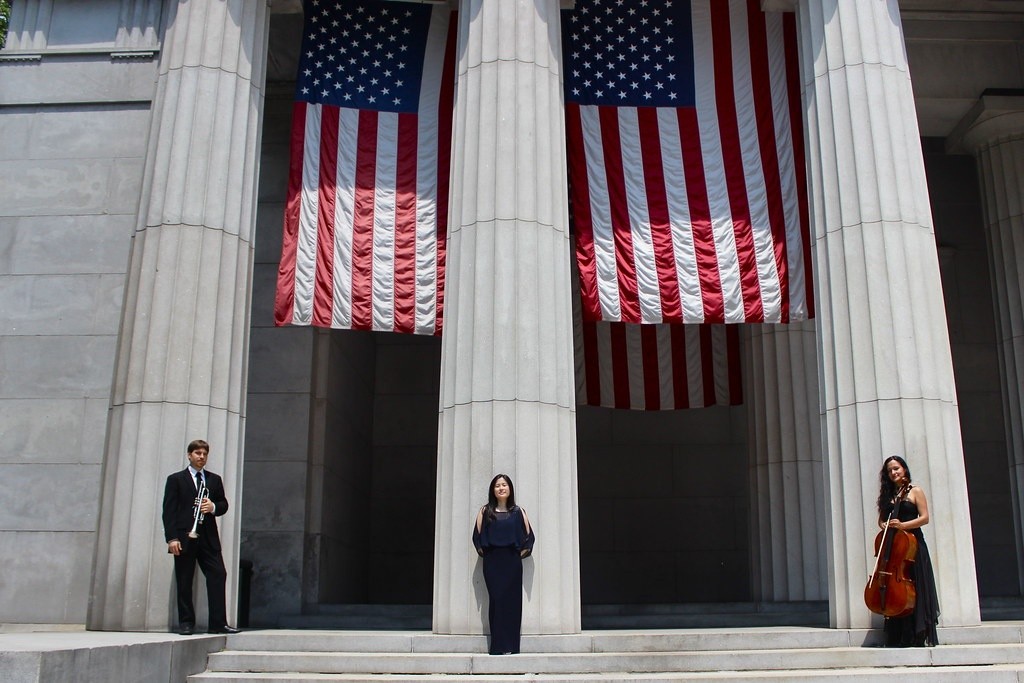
[208,625,240,633]
[179,622,192,635]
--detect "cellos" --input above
[862,476,918,648]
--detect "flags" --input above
[274,0,458,338]
[560,0,816,412]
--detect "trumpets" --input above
[187,478,210,539]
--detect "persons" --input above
[162,440,242,635]
[473,474,535,656]
[878,455,941,648]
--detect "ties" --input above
[196,471,204,492]
